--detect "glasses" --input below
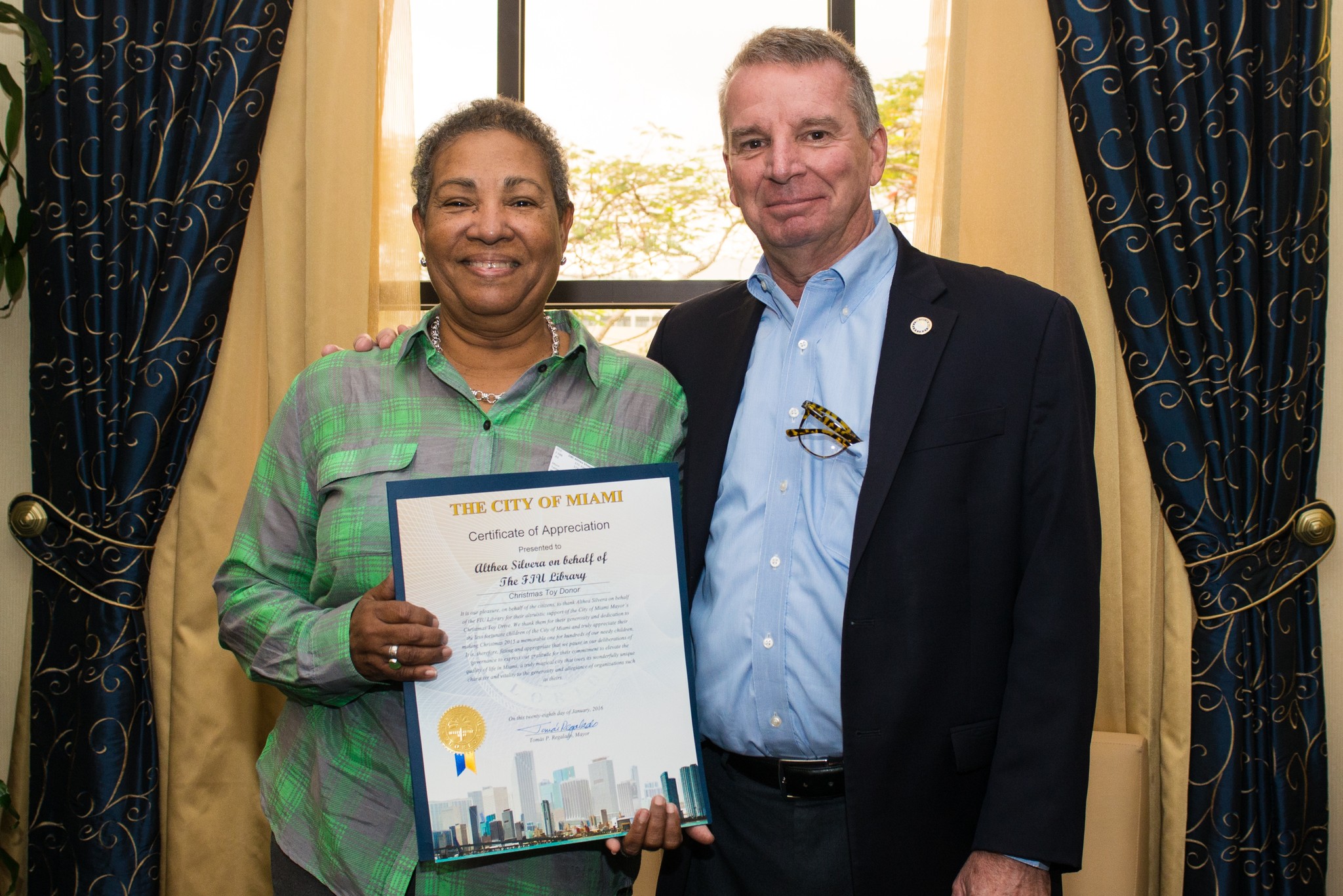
[787,401,861,460]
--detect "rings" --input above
[387,645,402,670]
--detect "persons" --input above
[212,101,719,896]
[320,24,1106,896]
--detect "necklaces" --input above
[427,310,559,406]
